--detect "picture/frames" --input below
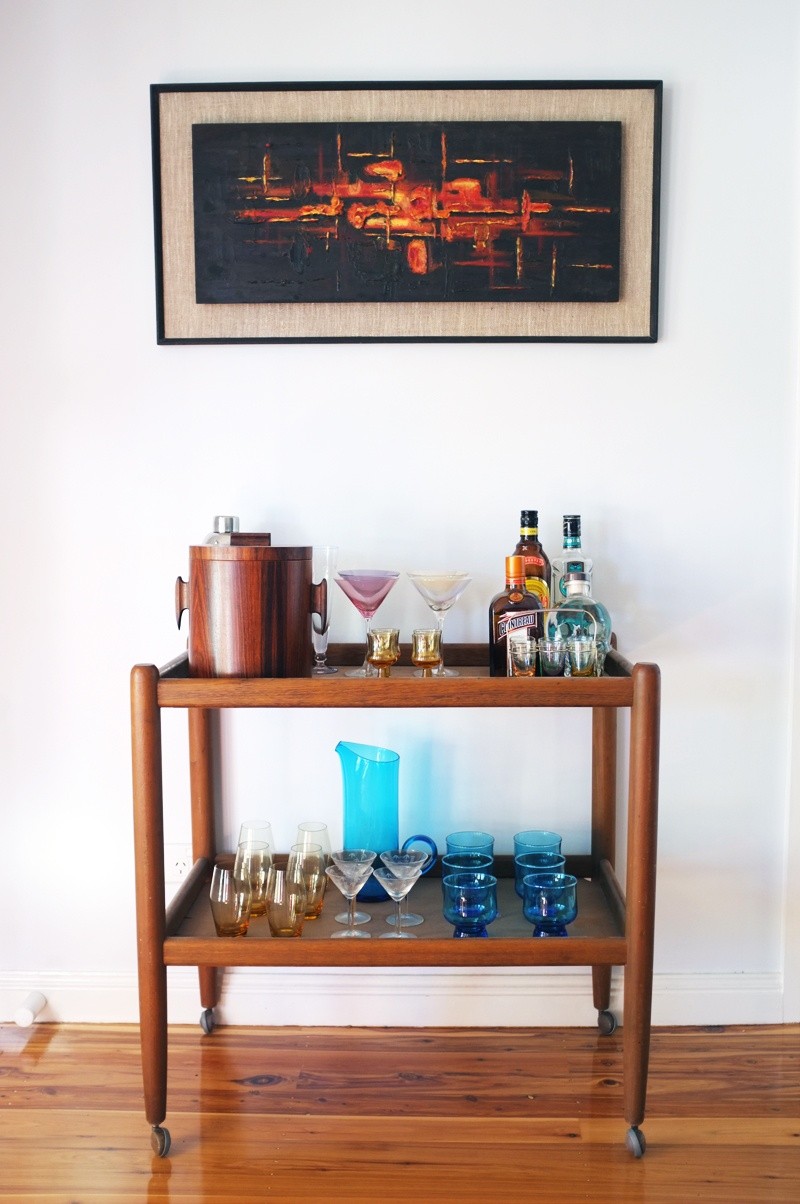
[150,80,662,344]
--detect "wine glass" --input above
[441,873,498,938]
[334,570,401,677]
[406,567,472,678]
[522,873,578,937]
[325,849,429,939]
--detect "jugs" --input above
[335,741,438,902]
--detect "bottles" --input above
[489,555,544,677]
[206,516,235,546]
[552,514,592,607]
[511,511,552,635]
[546,571,611,676]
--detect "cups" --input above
[264,861,307,938]
[510,635,598,677]
[233,841,275,918]
[446,831,495,856]
[209,861,252,937]
[514,852,567,905]
[441,852,494,879]
[514,830,562,857]
[297,822,331,892]
[238,822,274,863]
[285,843,326,920]
